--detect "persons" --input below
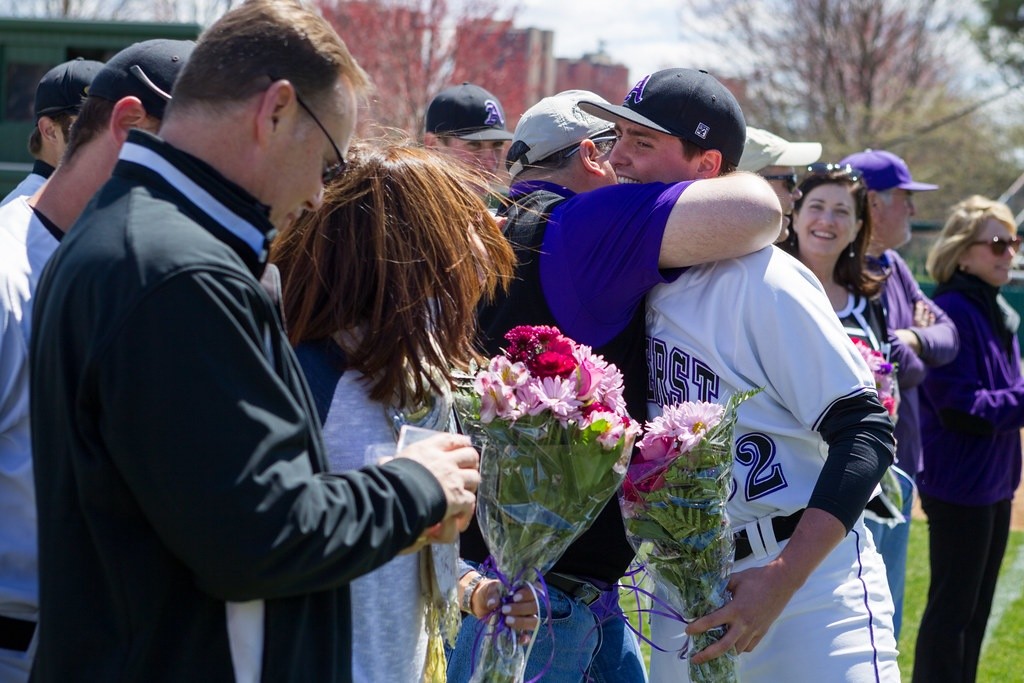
[910,196,1024,683]
[28,0,482,681]
[576,67,901,683]
[0,0,1024,683]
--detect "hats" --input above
[506,90,616,179]
[736,126,822,172]
[34,57,105,127]
[834,149,940,192]
[577,67,746,166]
[425,81,514,141]
[89,39,200,121]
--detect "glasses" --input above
[965,237,1021,257]
[805,162,863,182]
[761,175,798,193]
[267,72,347,183]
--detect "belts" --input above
[0,614,40,652]
[538,570,604,607]
[732,508,807,561]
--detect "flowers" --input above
[850,337,902,509]
[618,384,766,683]
[460,325,643,683]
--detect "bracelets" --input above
[462,574,482,617]
